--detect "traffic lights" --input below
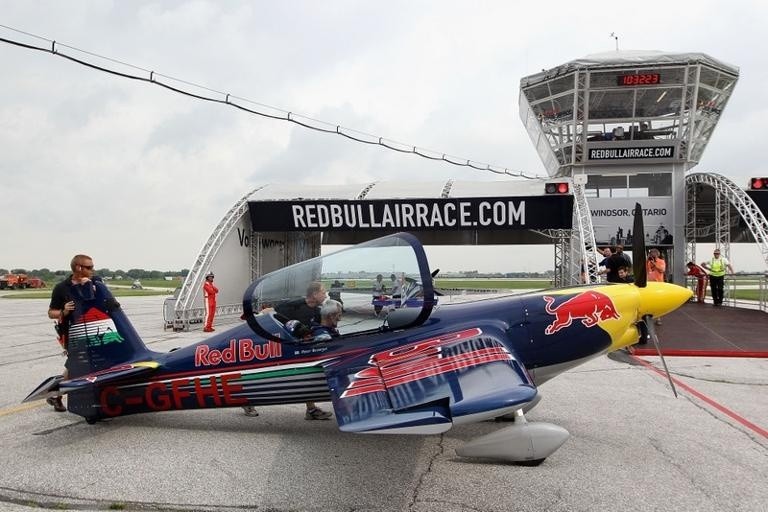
[749,176,768,190]
[544,182,571,194]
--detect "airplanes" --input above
[22,197,695,470]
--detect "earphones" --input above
[80,267,81,271]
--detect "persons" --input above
[238,281,334,421]
[328,280,346,314]
[312,299,343,342]
[609,123,650,140]
[45,255,103,412]
[701,248,736,306]
[391,274,403,308]
[372,274,385,317]
[580,242,667,345]
[202,270,219,332]
[683,262,707,305]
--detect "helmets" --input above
[205,270,214,277]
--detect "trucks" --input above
[0,274,30,290]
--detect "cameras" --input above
[648,249,655,260]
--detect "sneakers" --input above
[655,320,663,326]
[46,396,67,412]
[304,408,333,420]
[242,406,259,417]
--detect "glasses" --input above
[80,265,93,270]
